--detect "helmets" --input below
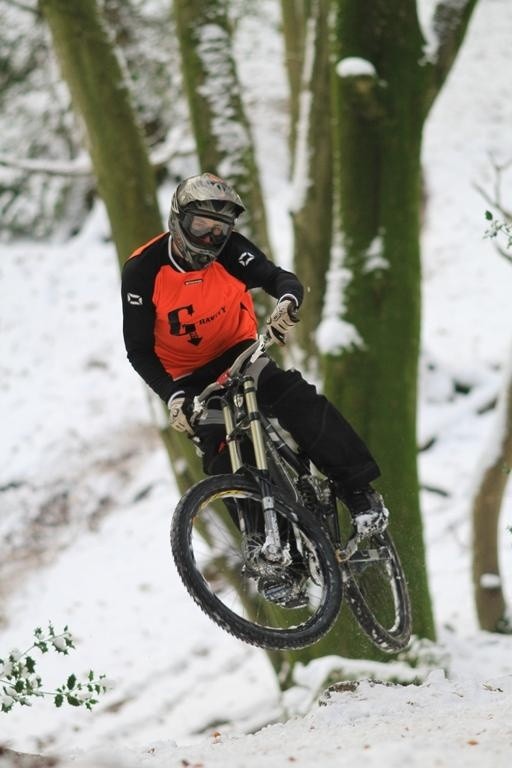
[169,173,245,270]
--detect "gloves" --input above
[266,294,301,345]
[168,396,200,435]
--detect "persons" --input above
[121,170,391,611]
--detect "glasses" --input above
[179,208,235,251]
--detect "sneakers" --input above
[258,576,309,609]
[347,487,388,538]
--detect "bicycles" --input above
[165,298,415,654]
